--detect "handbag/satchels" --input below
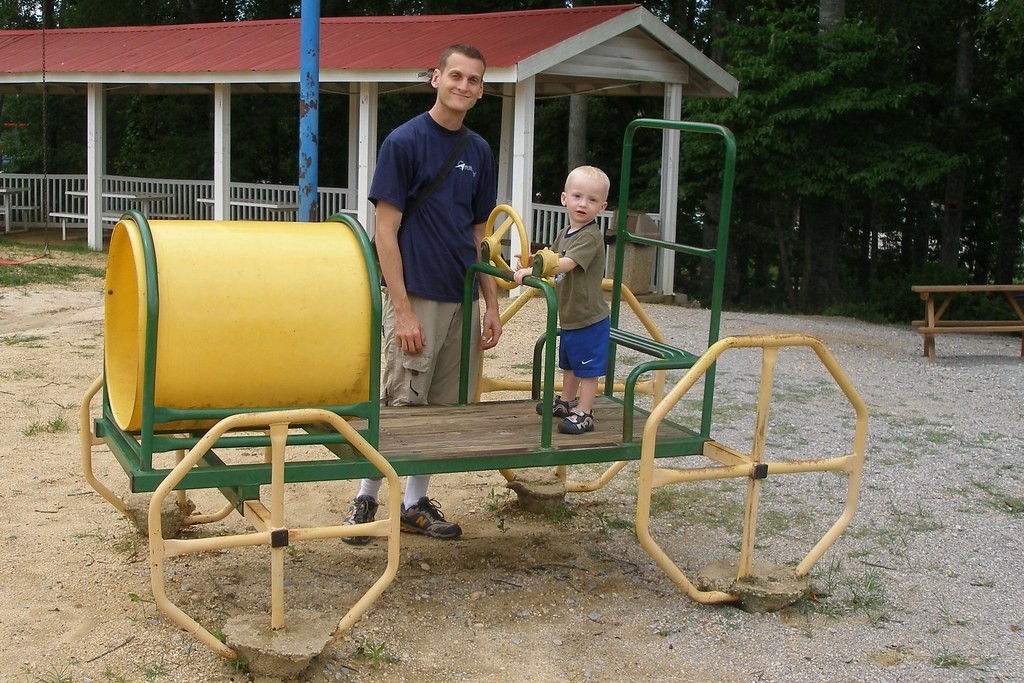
[371,235,379,262]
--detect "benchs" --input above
[911,320,1024,363]
[1,204,40,234]
[49,213,190,240]
[532,326,699,443]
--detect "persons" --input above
[341,44,503,545]
[513,165,610,433]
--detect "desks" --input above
[0,187,30,206]
[64,191,175,219]
[196,198,299,221]
[912,285,1024,328]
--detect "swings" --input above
[0,0,50,265]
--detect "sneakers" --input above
[400,497,462,539]
[341,495,378,544]
[558,408,594,433]
[536,395,578,418]
